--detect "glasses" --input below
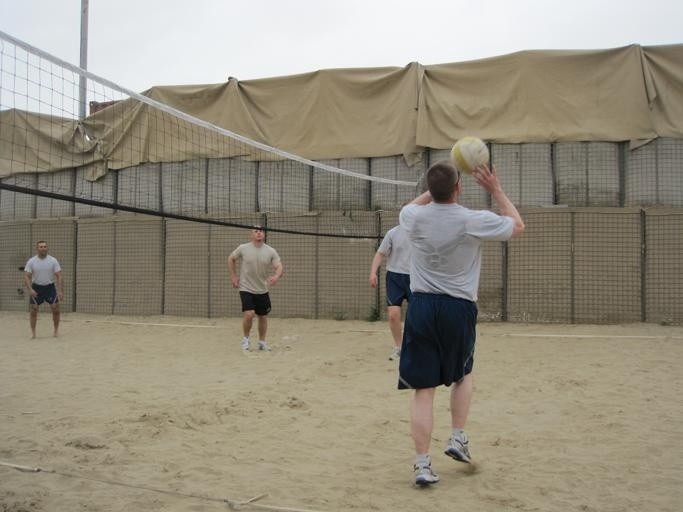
[455,170,461,186]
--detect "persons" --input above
[228,225,283,352]
[23,240,64,342]
[397,159,526,486]
[369,202,412,362]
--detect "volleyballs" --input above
[452,137,492,174]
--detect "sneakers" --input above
[241,337,250,351]
[442,433,471,464]
[412,460,440,485]
[258,340,272,352]
[389,346,401,361]
